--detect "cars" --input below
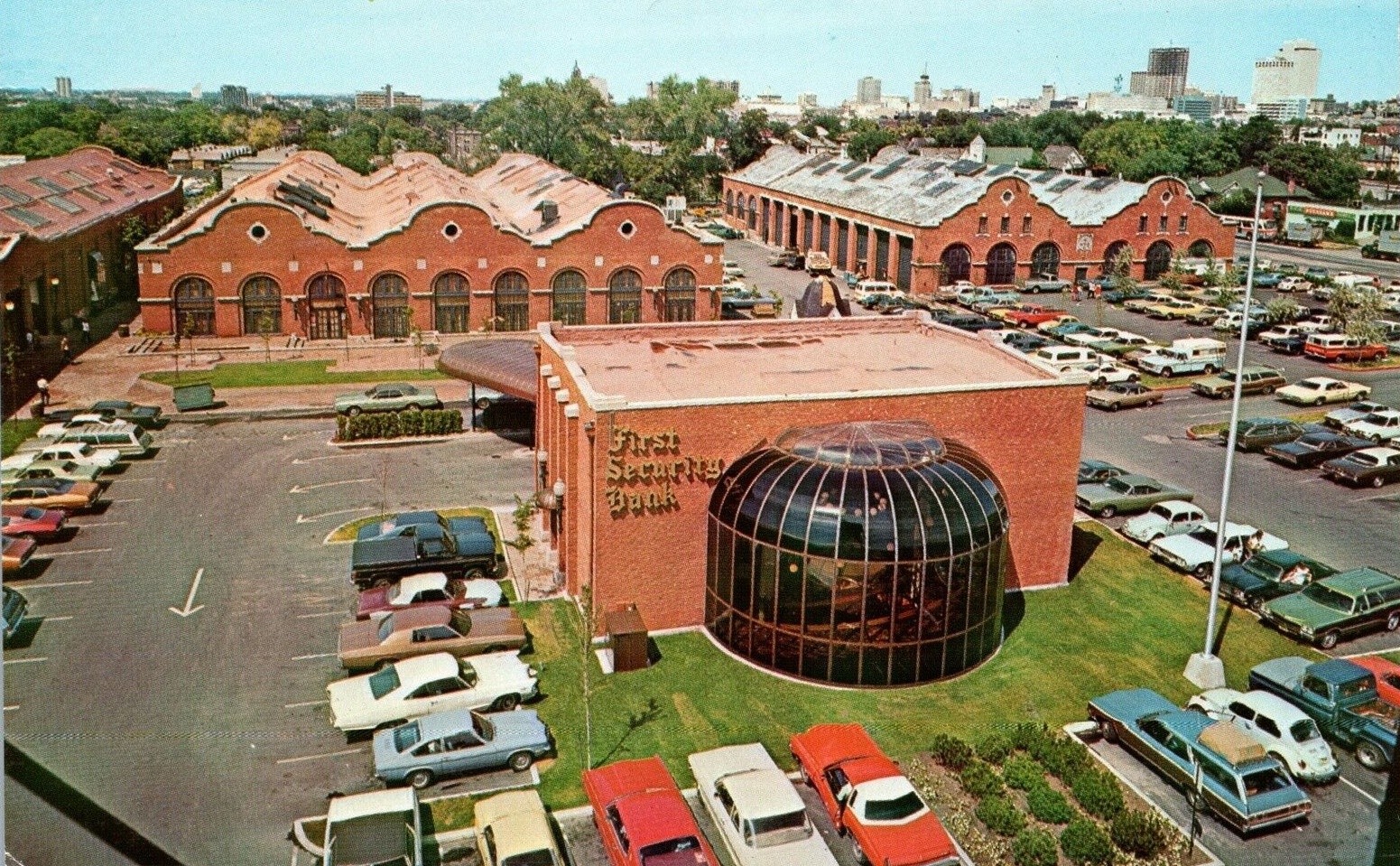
[580,754,722,866]
[1345,653,1400,711]
[354,570,504,624]
[372,705,552,789]
[843,254,1400,580]
[787,721,961,866]
[337,601,533,676]
[465,385,504,411]
[687,201,747,240]
[357,509,488,543]
[688,741,841,866]
[325,650,542,732]
[1087,688,1313,833]
[293,784,425,866]
[2,399,165,643]
[473,789,567,866]
[720,246,833,312]
[334,381,439,418]
[1200,548,1340,613]
[1259,565,1400,650]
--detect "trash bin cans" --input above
[172,383,216,412]
[118,324,130,337]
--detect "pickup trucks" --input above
[349,521,498,594]
[1249,655,1400,772]
[1187,687,1341,789]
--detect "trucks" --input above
[1360,228,1400,264]
[1274,220,1326,249]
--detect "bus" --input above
[1222,214,1278,242]
